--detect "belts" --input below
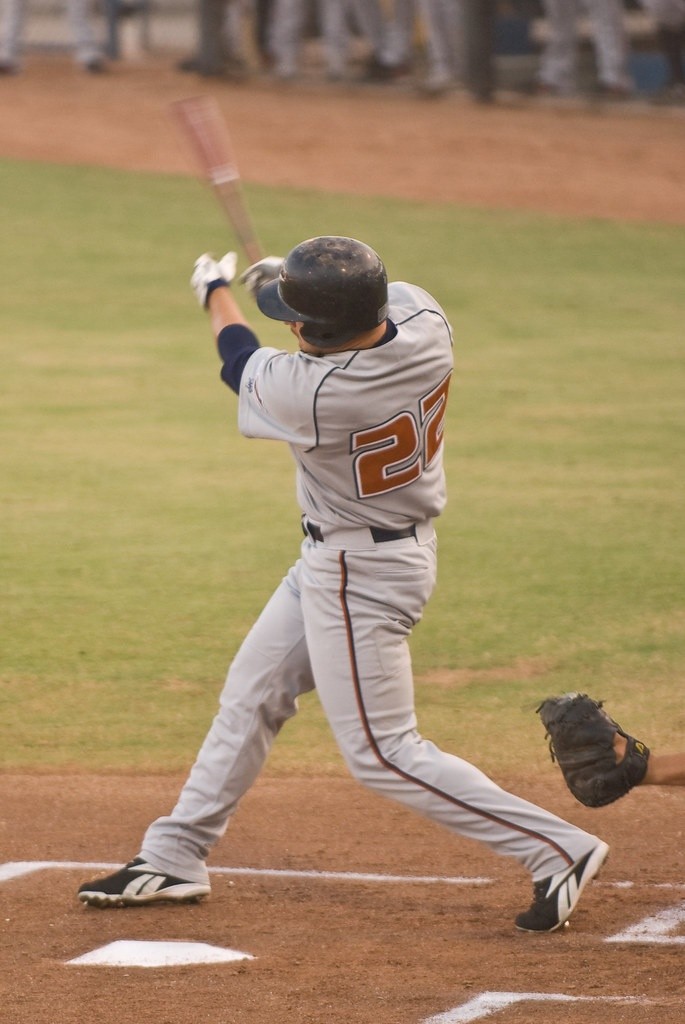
[302,519,417,543]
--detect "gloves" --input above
[239,256,286,298]
[191,251,237,313]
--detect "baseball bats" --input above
[171,89,265,264]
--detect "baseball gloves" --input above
[536,690,651,809]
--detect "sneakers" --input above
[78,854,211,909]
[513,835,613,934]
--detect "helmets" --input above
[256,236,389,348]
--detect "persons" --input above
[537,695,685,807]
[0,0,685,104]
[76,237,613,934]
